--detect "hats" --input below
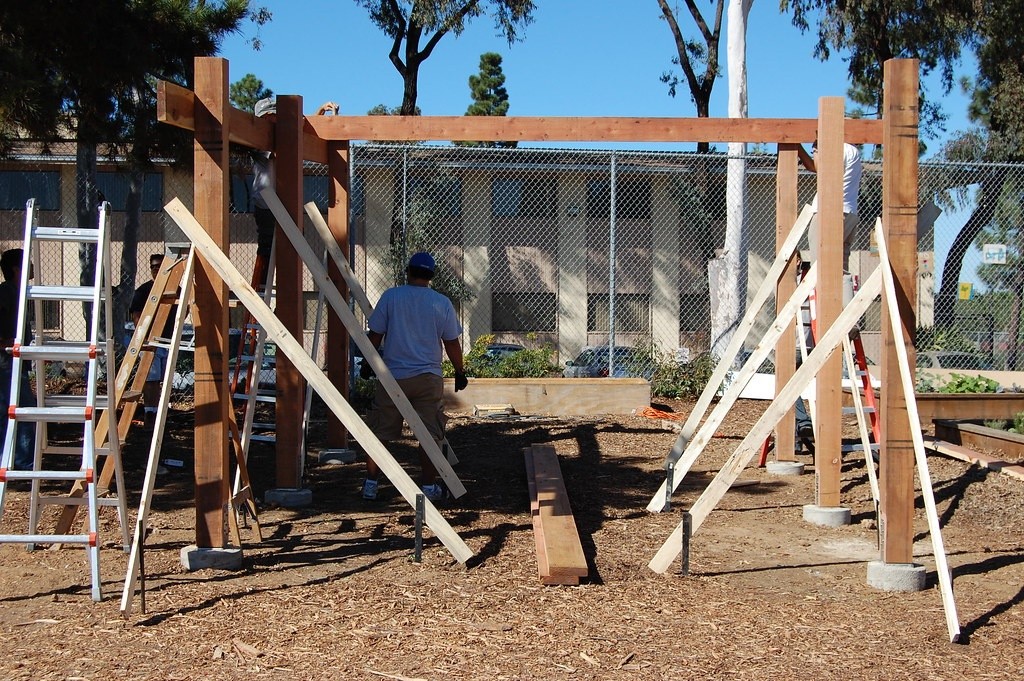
[404,251,435,272]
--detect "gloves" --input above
[360,366,376,381]
[455,371,468,392]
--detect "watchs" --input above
[456,368,466,374]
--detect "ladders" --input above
[795,252,901,556]
[1,197,326,602]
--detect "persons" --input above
[356,252,468,500]
[793,251,813,452]
[250,97,339,295]
[0,249,38,473]
[795,117,867,348]
[130,254,190,440]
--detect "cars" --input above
[916,350,996,369]
[28,320,380,391]
[739,345,878,381]
[561,346,661,381]
[468,343,528,360]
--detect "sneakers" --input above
[362,479,379,500]
[422,483,451,505]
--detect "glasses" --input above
[150,264,160,269]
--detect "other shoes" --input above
[144,411,156,432]
[15,483,31,491]
